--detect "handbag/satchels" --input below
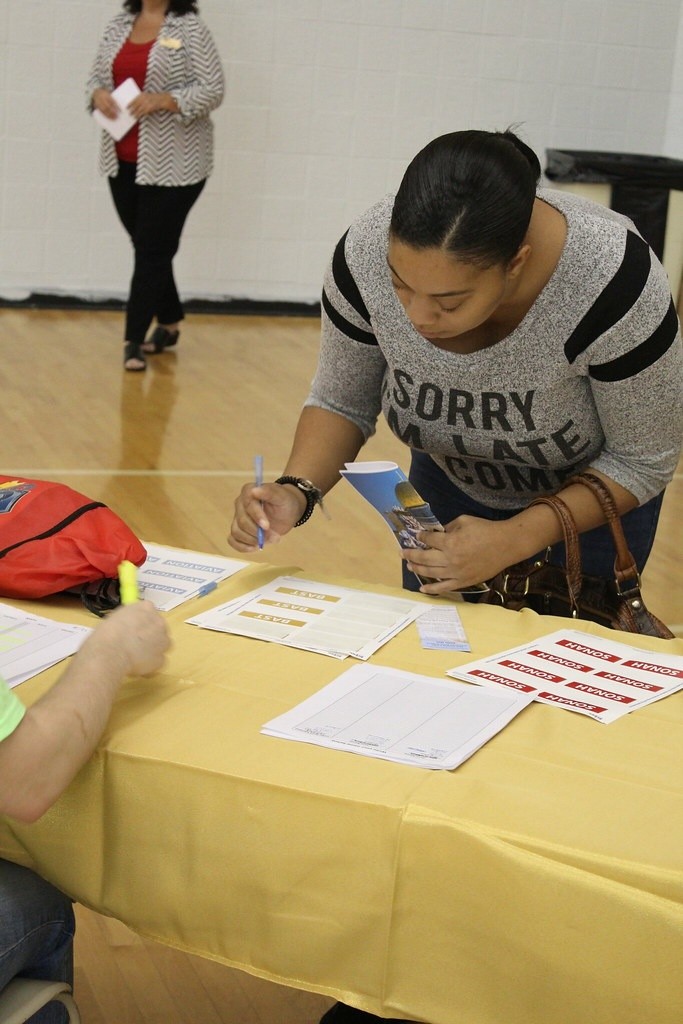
[460,474,674,641]
[0,474,147,617]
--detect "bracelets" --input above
[273,475,314,527]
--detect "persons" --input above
[0,599,172,1024]
[227,121,683,1024]
[85,0,224,371]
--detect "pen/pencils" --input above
[254,454,265,552]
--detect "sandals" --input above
[123,341,147,371]
[141,327,180,355]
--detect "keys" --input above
[313,487,331,520]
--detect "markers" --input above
[117,559,140,604]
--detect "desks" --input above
[0,537,681,1024]
